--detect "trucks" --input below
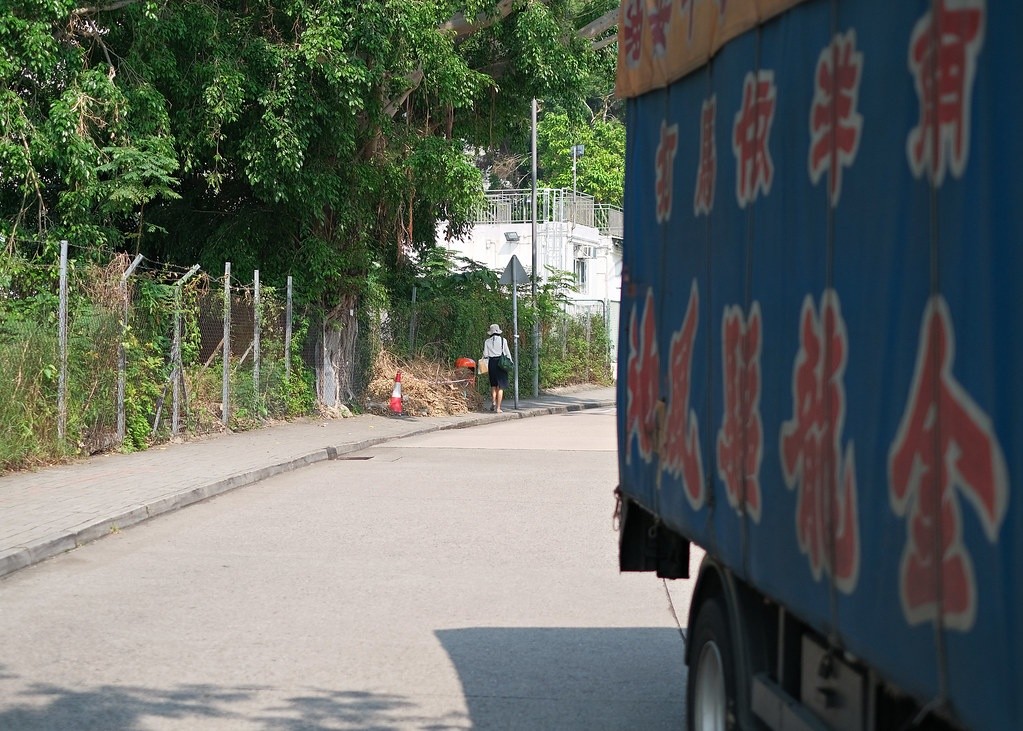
[611,0,1020,727]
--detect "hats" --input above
[486,324,503,336]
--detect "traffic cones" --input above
[390,371,403,416]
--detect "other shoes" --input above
[496,410,502,413]
[490,403,496,411]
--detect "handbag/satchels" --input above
[477,355,487,375]
[498,353,512,374]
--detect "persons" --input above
[483,324,513,413]
